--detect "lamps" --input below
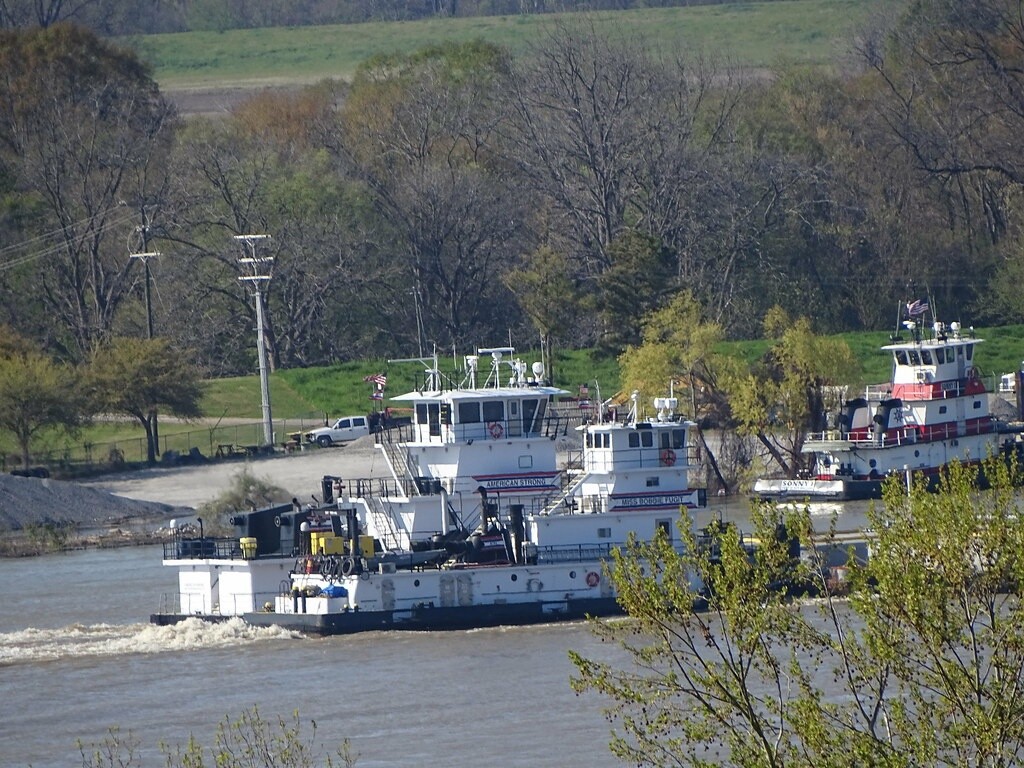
[551,435,557,440]
[678,416,685,424]
[467,439,473,445]
[951,440,959,446]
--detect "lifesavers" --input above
[586,571,600,587]
[662,449,676,465]
[489,423,504,440]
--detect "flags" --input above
[363,372,387,386]
[908,298,928,315]
[369,390,384,401]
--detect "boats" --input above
[149,327,882,634]
[754,284,1024,504]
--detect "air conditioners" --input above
[916,371,931,379]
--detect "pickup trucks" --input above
[305,415,411,448]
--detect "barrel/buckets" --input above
[240,537,257,559]
